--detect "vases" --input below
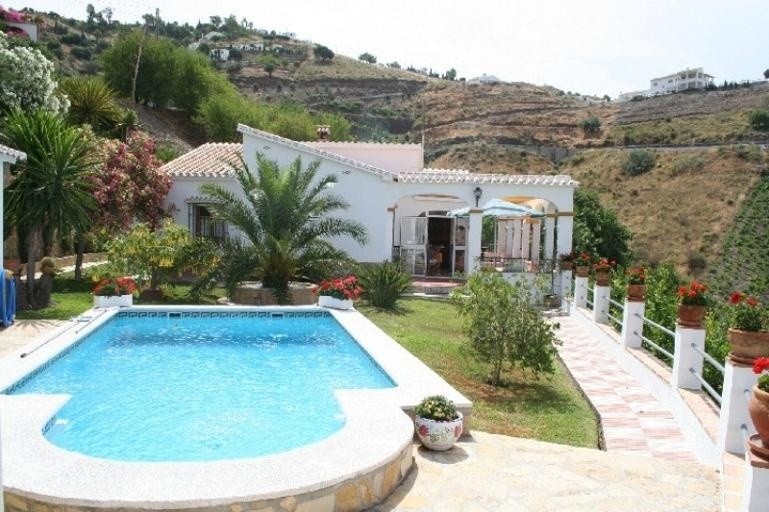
[318,295,353,310]
[727,328,769,365]
[596,273,610,285]
[576,264,589,277]
[628,284,645,300]
[749,384,769,458]
[94,295,133,306]
[562,262,572,269]
[676,304,705,326]
[414,412,464,451]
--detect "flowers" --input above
[753,356,769,393]
[593,258,617,272]
[92,277,137,297]
[678,283,707,306]
[576,252,590,265]
[416,396,457,422]
[627,267,646,284]
[731,293,766,332]
[311,275,362,300]
[560,254,575,264]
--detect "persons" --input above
[429,247,444,276]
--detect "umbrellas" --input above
[443,197,546,270]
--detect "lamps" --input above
[473,187,482,207]
